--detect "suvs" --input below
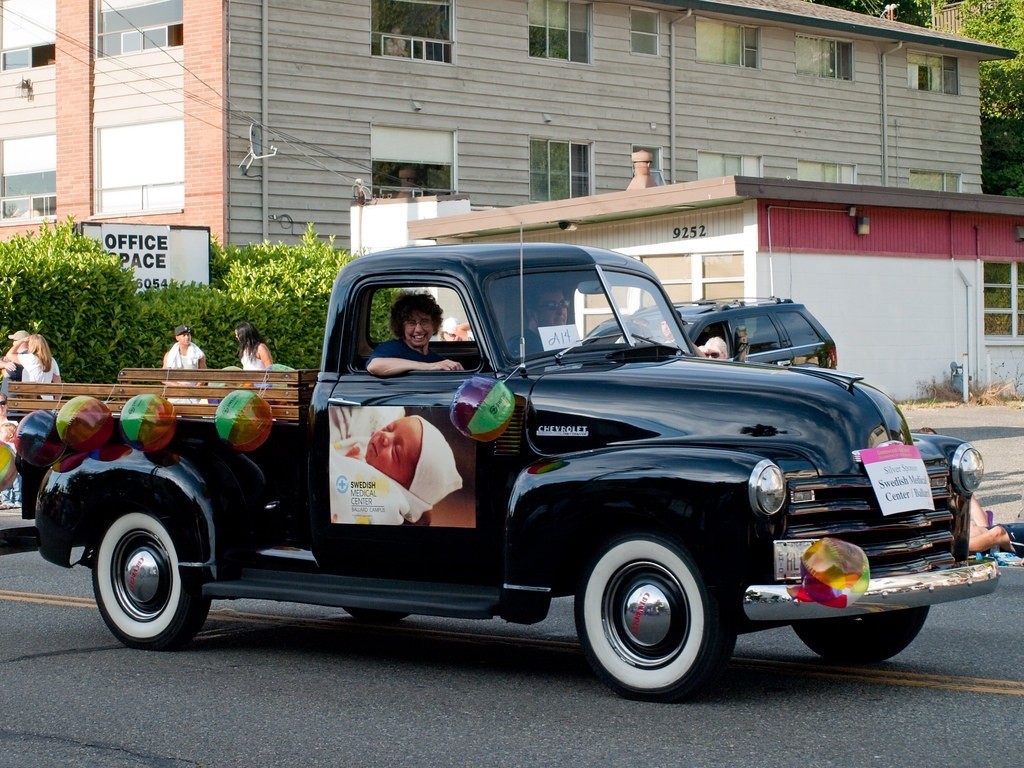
[580,295,838,369]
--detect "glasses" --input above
[544,299,568,310]
[403,320,434,328]
[0,401,6,405]
[704,352,723,359]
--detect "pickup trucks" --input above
[5,241,1000,704]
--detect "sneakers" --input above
[8,502,14,509]
[14,502,22,507]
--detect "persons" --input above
[697,336,728,360]
[384,27,409,57]
[161,325,208,403]
[614,317,675,343]
[440,317,473,341]
[507,280,568,358]
[234,322,272,387]
[0,330,62,519]
[365,292,471,377]
[330,406,463,525]
[917,427,1024,567]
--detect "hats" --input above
[8,330,30,341]
[675,309,688,325]
[409,415,462,505]
[175,324,191,336]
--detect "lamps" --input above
[410,101,421,110]
[1014,226,1024,242]
[543,114,552,123]
[857,216,870,235]
[15,75,31,98]
[558,221,578,232]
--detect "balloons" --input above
[120,393,176,452]
[215,390,272,452]
[14,395,113,467]
[449,374,517,442]
[799,536,870,610]
